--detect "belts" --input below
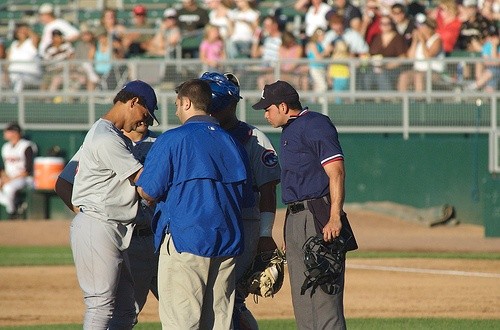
[289,203,305,214]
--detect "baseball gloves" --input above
[244,239,285,299]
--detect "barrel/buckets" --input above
[33,157,66,190]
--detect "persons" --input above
[55,123,159,307]
[252,80,348,330]
[0,122,39,220]
[135,79,256,330]
[69,80,160,330]
[200,71,282,330]
[0,0,500,104]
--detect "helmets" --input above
[199,71,240,115]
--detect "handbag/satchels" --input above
[307,193,358,252]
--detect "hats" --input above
[122,79,160,127]
[163,8,178,18]
[38,2,54,14]
[7,125,20,130]
[252,82,299,110]
[462,0,477,8]
[134,6,145,14]
[54,30,62,35]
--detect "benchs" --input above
[19,189,58,219]
